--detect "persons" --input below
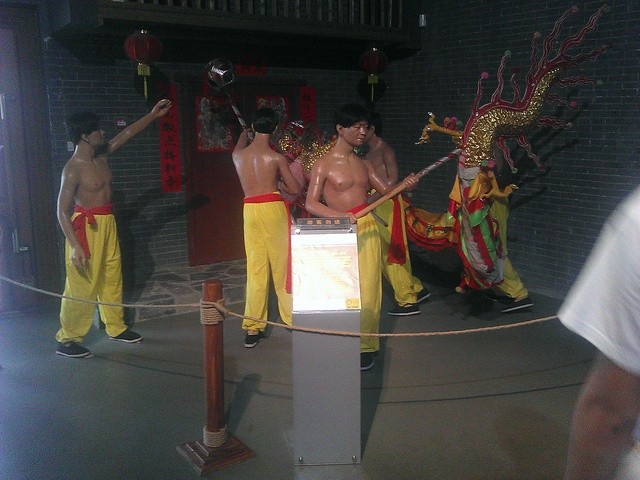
[305,98,418,371]
[556,179,640,480]
[55,98,172,358]
[354,113,432,316]
[457,136,535,313]
[232,106,300,347]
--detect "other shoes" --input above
[56,342,91,357]
[502,298,534,313]
[110,330,143,343]
[416,288,431,302]
[244,332,265,347]
[387,306,421,315]
[486,292,498,302]
[360,352,375,370]
